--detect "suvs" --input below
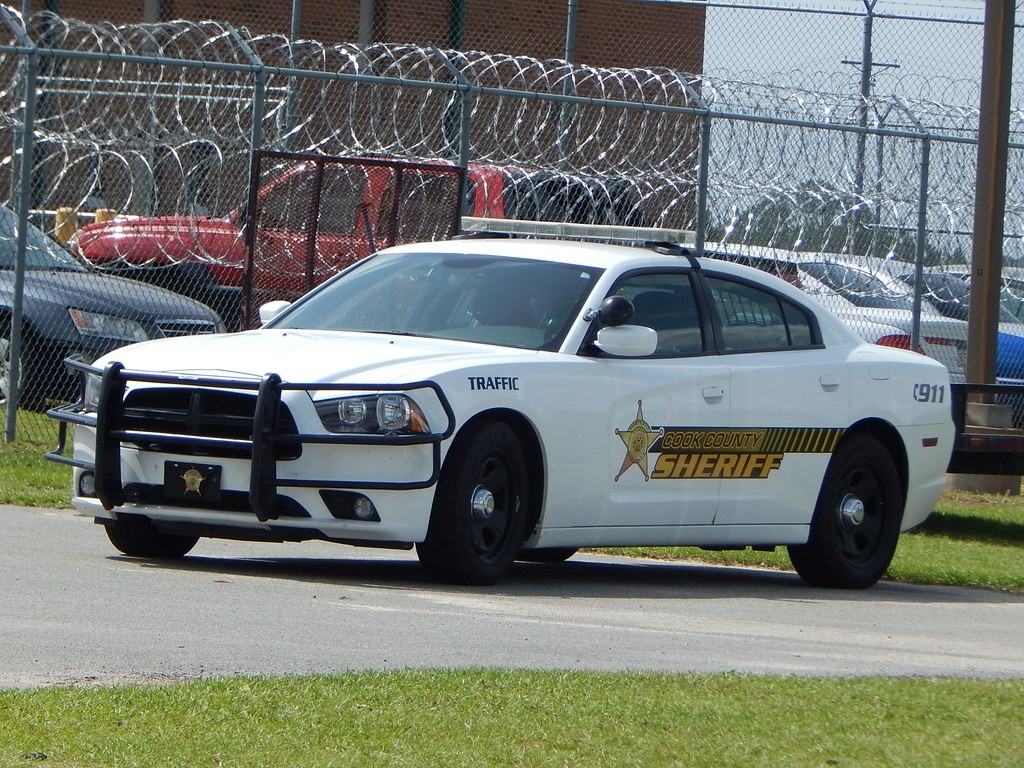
[67,155,678,334]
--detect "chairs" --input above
[471,283,703,353]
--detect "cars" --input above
[675,234,1024,476]
[0,202,230,415]
[24,208,141,247]
[44,210,958,586]
